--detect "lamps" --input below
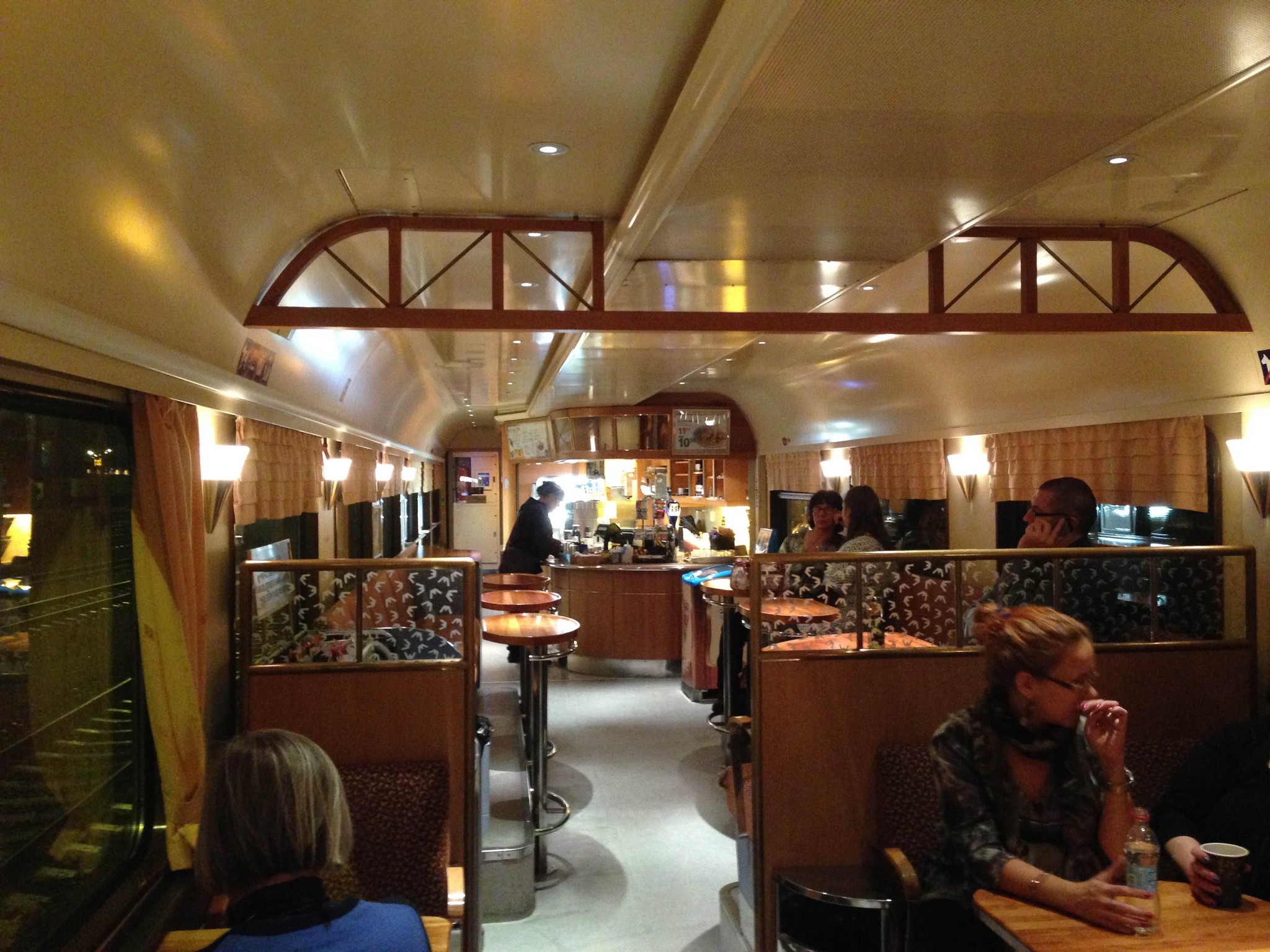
[323,457,352,510]
[1227,438,1270,520]
[375,463,395,500]
[818,456,842,496]
[401,467,417,496]
[945,454,977,506]
[203,444,251,535]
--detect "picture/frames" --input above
[503,416,554,464]
[673,408,730,456]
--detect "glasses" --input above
[811,505,837,514]
[1045,671,1101,694]
[1027,504,1076,518]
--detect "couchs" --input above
[311,544,482,680]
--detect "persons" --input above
[1151,702,1270,908]
[916,596,1159,934]
[982,476,1122,645]
[761,487,900,657]
[499,480,566,663]
[710,490,844,714]
[193,731,432,952]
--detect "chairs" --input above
[875,736,946,952]
[325,759,457,918]
[895,573,966,649]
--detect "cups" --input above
[564,524,595,546]
[1200,843,1247,909]
[678,487,689,496]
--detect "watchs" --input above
[1097,765,1136,795]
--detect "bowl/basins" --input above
[693,423,728,448]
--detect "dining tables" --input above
[974,880,1269,952]
[701,573,940,838]
[159,915,453,952]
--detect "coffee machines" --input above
[563,499,612,546]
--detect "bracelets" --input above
[1028,870,1052,906]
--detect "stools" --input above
[478,612,581,883]
[480,588,562,786]
[482,573,553,589]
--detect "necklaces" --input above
[808,531,831,550]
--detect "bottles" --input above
[867,596,887,650]
[1122,807,1162,935]
[730,558,750,591]
